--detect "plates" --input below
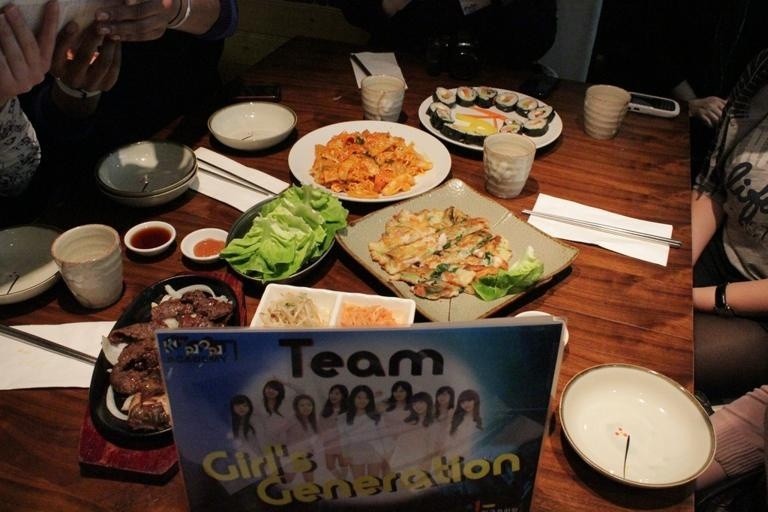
[207,101,298,150]
[224,192,335,281]
[418,87,563,151]
[179,228,231,262]
[249,283,417,338]
[0,224,62,304]
[559,362,718,488]
[87,273,236,447]
[124,221,176,257]
[287,120,453,204]
[95,141,195,208]
[333,177,582,321]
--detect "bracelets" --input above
[167,0,190,29]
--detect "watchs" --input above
[713,281,735,318]
[56,76,101,99]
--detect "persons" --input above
[433,387,456,428]
[255,375,306,428]
[0,0,60,209]
[390,392,442,472]
[446,389,484,454]
[338,0,559,64]
[20,0,239,204]
[694,384,768,512]
[319,384,349,481]
[336,385,392,478]
[690,45,768,403]
[279,393,320,482]
[227,395,265,451]
[380,381,412,478]
[602,0,768,138]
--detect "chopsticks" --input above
[2,319,98,372]
[521,207,684,250]
[192,157,281,197]
[348,50,375,77]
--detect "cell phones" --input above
[627,92,681,118]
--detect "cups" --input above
[49,222,126,319]
[582,84,631,143]
[361,75,404,122]
[482,132,535,200]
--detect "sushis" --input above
[477,86,497,108]
[456,86,478,106]
[495,92,519,113]
[467,129,487,146]
[500,125,521,136]
[426,102,452,113]
[503,118,525,132]
[430,108,453,130]
[515,97,538,118]
[433,85,457,108]
[521,119,549,137]
[440,120,466,143]
[527,105,555,123]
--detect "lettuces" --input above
[220,183,349,283]
[471,244,543,302]
